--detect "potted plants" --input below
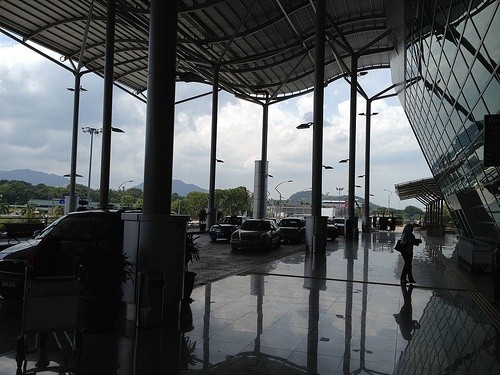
[73,238,138,335]
[184,232,202,299]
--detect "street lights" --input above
[383,187,393,211]
[274,180,293,216]
[337,187,345,218]
[81,125,101,209]
[117,179,134,196]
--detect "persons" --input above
[345,217,353,240]
[401,223,419,283]
[373,216,396,231]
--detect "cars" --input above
[230,218,281,250]
[278,218,305,242]
[326,220,339,242]
[394,214,403,227]
[334,218,346,233]
[209,215,246,241]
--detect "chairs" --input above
[16,265,89,375]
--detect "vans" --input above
[0,209,178,305]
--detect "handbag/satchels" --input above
[394,240,404,252]
